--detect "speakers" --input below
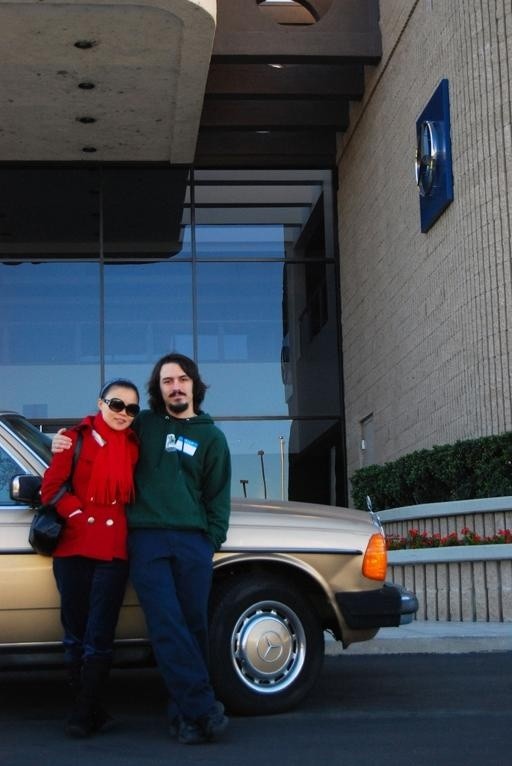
[59,653,118,740]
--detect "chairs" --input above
[200,700,230,743]
[177,713,206,744]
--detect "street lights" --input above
[28,427,84,557]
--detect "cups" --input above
[101,397,140,417]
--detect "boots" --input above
[0,412,417,717]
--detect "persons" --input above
[40,381,140,737]
[51,353,232,744]
